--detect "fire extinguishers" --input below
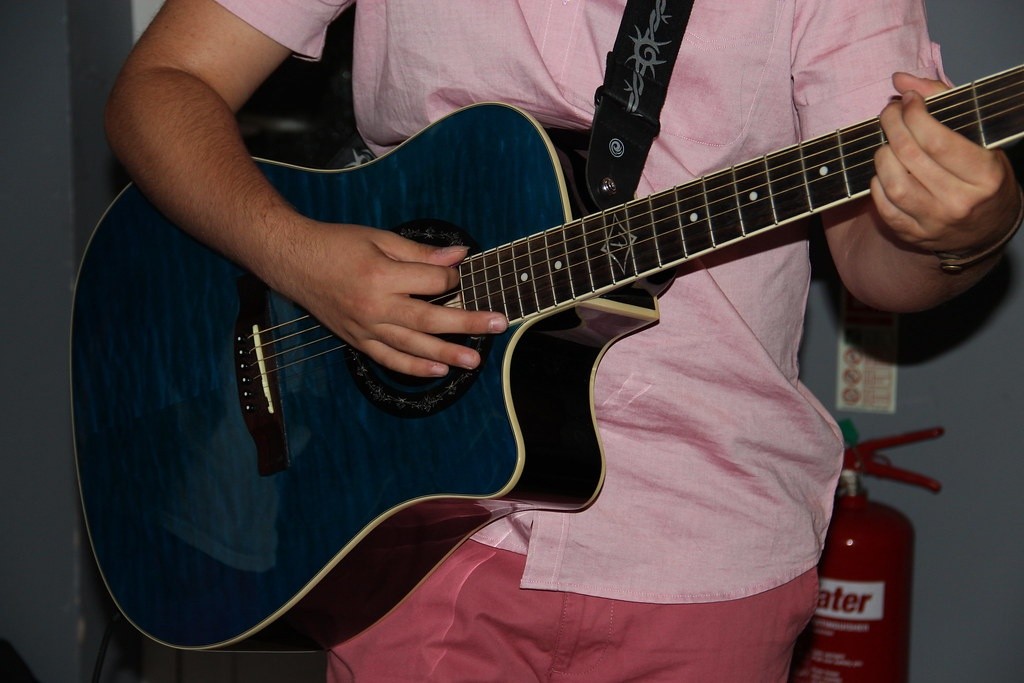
[788,426,944,683]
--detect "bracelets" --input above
[934,181,1024,274]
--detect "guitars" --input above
[66,64,1024,654]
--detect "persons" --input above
[103,1,1024,683]
